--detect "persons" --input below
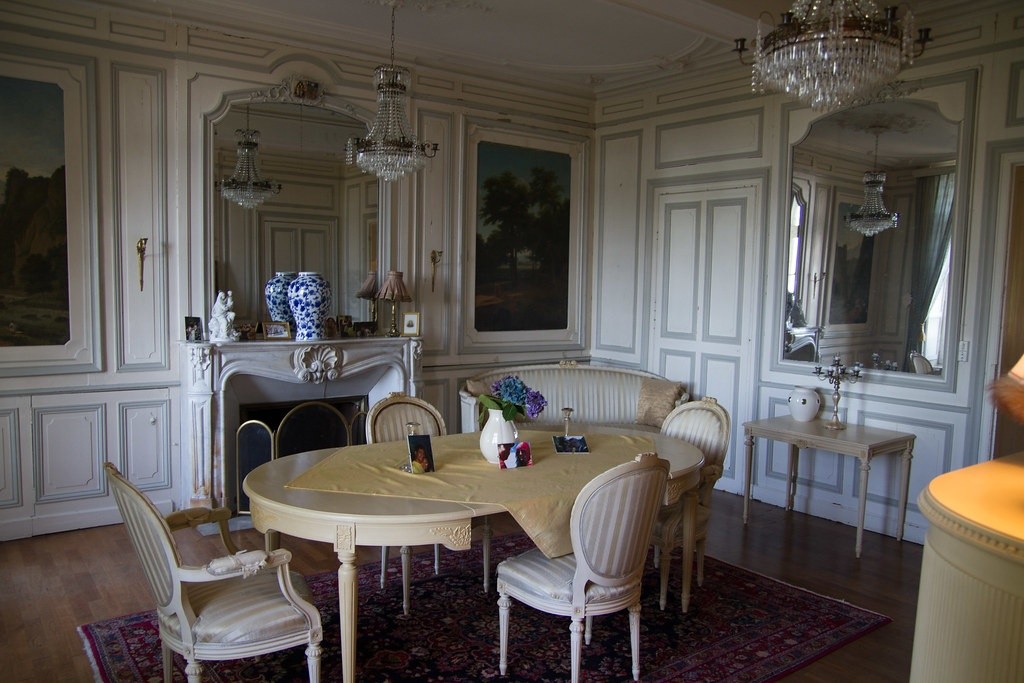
[340,316,352,337]
[498,442,532,469]
[412,447,428,474]
[268,324,286,335]
[210,289,241,340]
[556,436,589,452]
[186,322,202,340]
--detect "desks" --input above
[243,423,707,682]
[742,416,916,559]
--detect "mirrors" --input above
[768,64,983,393]
[208,73,379,336]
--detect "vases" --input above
[288,270,333,342]
[263,272,297,331]
[477,408,525,462]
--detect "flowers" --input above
[477,375,549,419]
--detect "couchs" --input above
[458,356,685,439]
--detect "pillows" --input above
[636,380,681,427]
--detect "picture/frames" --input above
[401,312,420,337]
[263,321,292,339]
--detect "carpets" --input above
[75,533,893,682]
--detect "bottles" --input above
[265,272,299,338]
[789,385,820,422]
[287,272,332,342]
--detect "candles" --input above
[874,352,897,365]
[817,355,861,371]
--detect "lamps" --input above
[345,0,440,183]
[836,108,901,240]
[358,272,381,322]
[378,272,411,337]
[219,103,281,210]
[737,0,933,112]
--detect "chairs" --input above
[105,461,324,683]
[496,452,672,681]
[365,397,491,616]
[908,350,933,373]
[651,396,729,610]
